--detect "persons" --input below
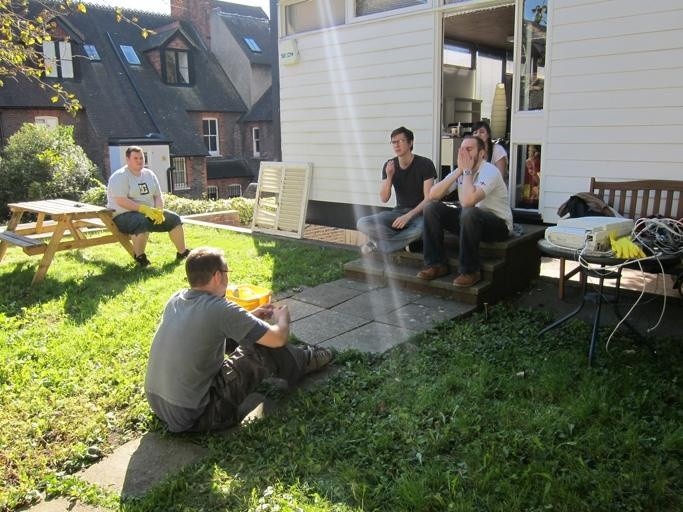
[144,246,339,433]
[355,127,438,255]
[415,135,515,288]
[107,145,191,267]
[472,121,509,183]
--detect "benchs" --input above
[0,198,137,286]
[537,176,683,299]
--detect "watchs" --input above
[463,169,472,177]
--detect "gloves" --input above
[138,205,165,225]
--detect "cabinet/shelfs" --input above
[441,96,482,171]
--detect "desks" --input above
[537,236,683,371]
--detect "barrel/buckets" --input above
[227,282,272,312]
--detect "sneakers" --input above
[416,265,448,280]
[452,272,482,288]
[300,346,333,376]
[406,243,423,253]
[361,239,375,254]
[176,250,189,258]
[134,253,151,266]
